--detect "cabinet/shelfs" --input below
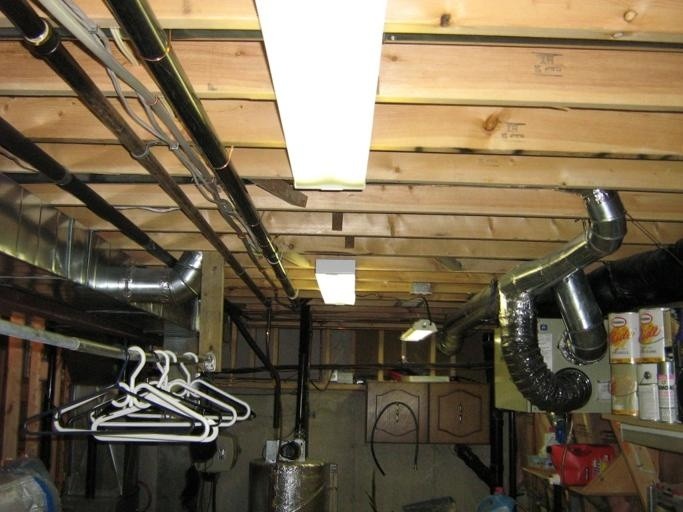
[366,380,428,444]
[428,382,490,444]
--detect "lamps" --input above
[254,2,385,192]
[316,259,356,305]
[399,282,438,342]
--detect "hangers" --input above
[21,347,255,443]
